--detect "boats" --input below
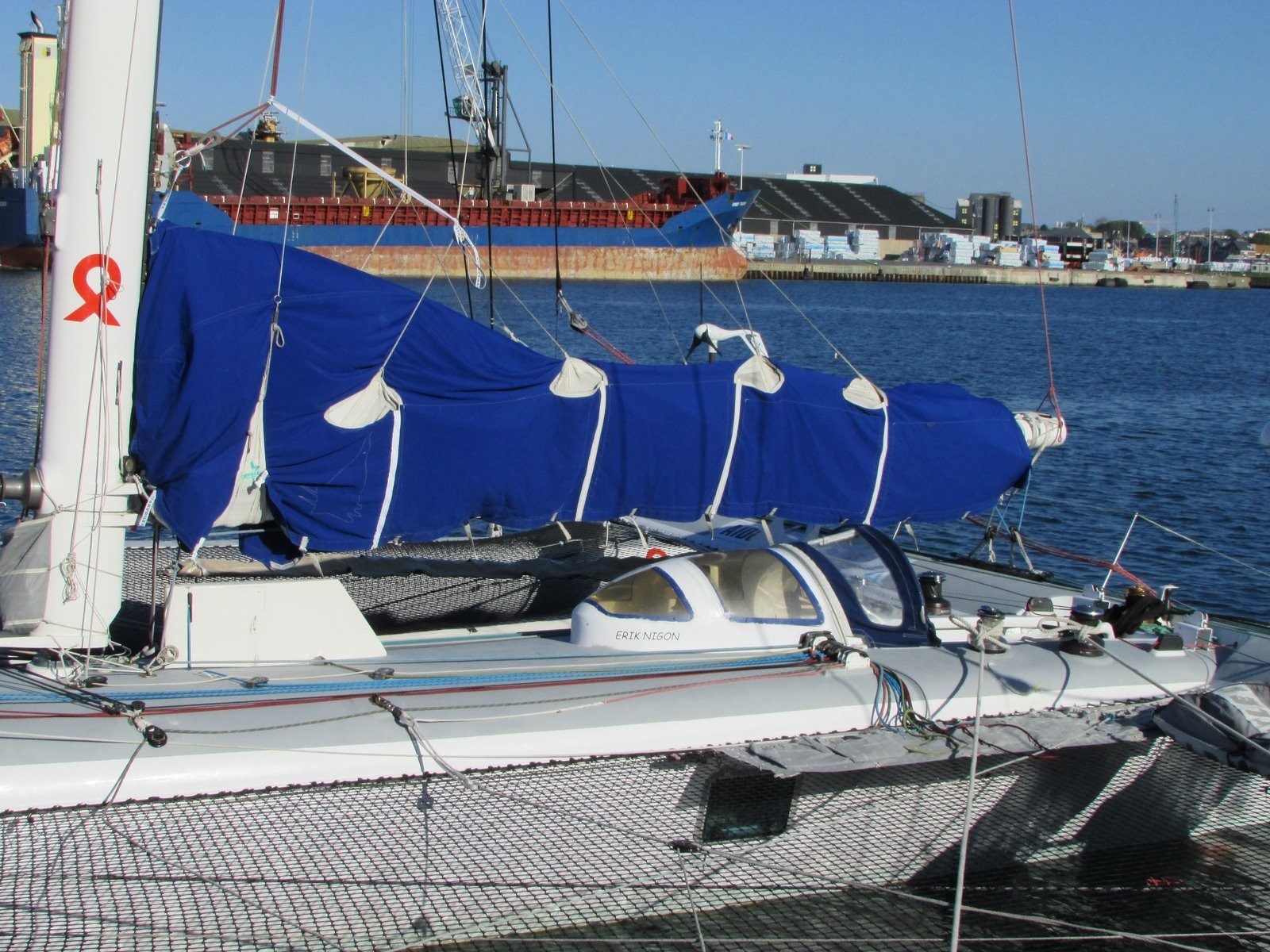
[1,1,762,282]
[0,191,1219,814]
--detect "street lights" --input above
[1154,213,1161,258]
[735,145,753,232]
[1207,208,1215,261]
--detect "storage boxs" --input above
[897,233,1064,269]
[732,229,880,260]
[1082,248,1124,272]
